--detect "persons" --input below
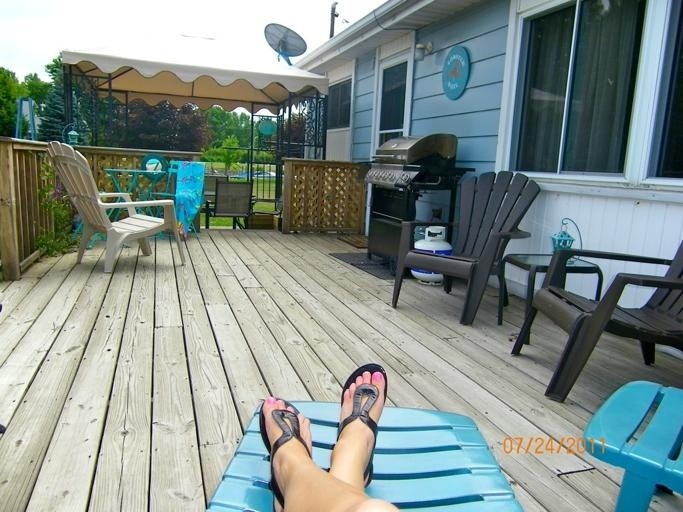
[259,362,401,512]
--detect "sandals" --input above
[335,363,388,488]
[259,397,313,512]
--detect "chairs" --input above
[391,170,539,326]
[46,140,283,273]
[585,381,683,512]
[204,401,521,511]
[513,241,682,403]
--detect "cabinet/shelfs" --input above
[369,164,476,276]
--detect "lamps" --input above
[415,40,434,61]
